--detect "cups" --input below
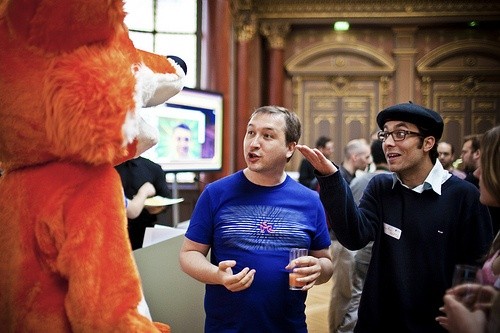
[452,265,483,312]
[289,248,308,290]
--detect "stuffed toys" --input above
[0,0,187,333]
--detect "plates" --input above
[144,196,184,208]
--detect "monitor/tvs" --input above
[140,87,224,173]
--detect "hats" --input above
[376,101,444,140]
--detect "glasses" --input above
[378,130,420,142]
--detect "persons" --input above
[113,155,170,251]
[436,124,500,333]
[328,130,395,333]
[297,137,334,189]
[320,139,371,232]
[179,105,334,333]
[295,101,494,333]
[161,123,198,162]
[124,182,156,219]
